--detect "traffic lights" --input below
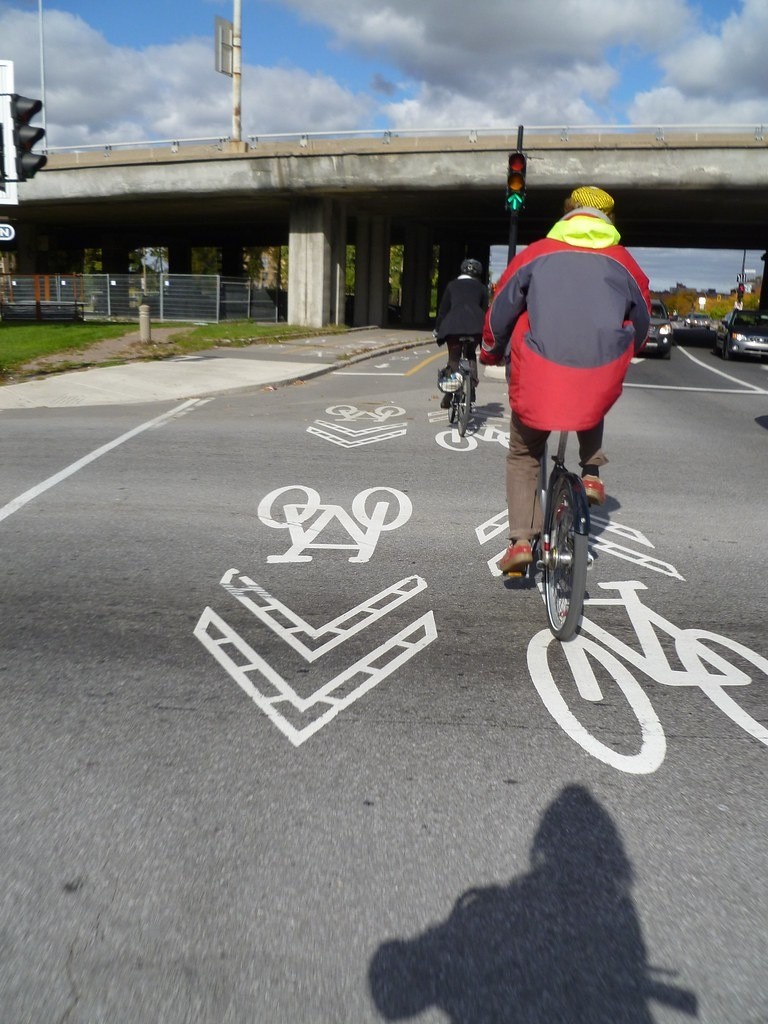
[506,146,525,211]
[737,283,744,301]
[9,93,46,187]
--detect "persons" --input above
[483,185,650,573]
[432,259,489,410]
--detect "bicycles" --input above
[432,325,480,441]
[479,347,596,640]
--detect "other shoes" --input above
[495,540,533,572]
[581,474,605,505]
[440,394,452,408]
[471,388,475,402]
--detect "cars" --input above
[683,313,713,330]
[637,298,674,358]
[715,308,768,363]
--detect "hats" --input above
[462,259,483,277]
[570,185,615,214]
